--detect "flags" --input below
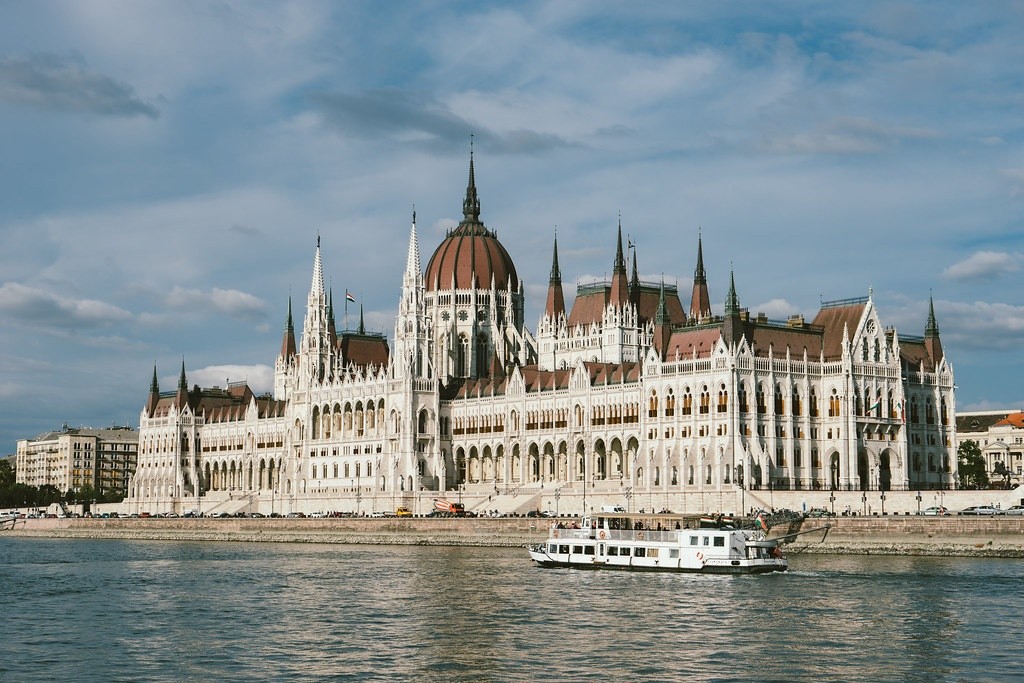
[347,294,354,302]
[898,397,905,423]
[866,397,881,414]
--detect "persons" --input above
[716,511,720,515]
[868,505,871,515]
[550,506,690,531]
[325,510,365,518]
[482,507,541,518]
[750,506,784,518]
[845,505,852,516]
[939,505,945,517]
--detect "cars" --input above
[921,505,1024,515]
[528,511,546,517]
[543,510,557,518]
[810,508,828,516]
[0,511,326,518]
[371,512,385,518]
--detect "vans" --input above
[397,507,412,518]
[600,506,625,514]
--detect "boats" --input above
[529,513,787,574]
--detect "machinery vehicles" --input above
[432,499,464,518]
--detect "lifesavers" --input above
[552,529,558,537]
[598,529,606,539]
[635,531,645,542]
[694,551,706,561]
